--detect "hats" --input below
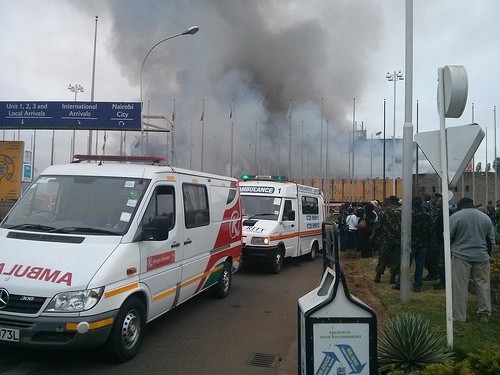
[431,192,443,197]
[389,196,402,206]
[370,200,378,208]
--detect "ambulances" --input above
[0,155,242,363]
[238,173,327,275]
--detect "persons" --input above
[443,198,495,323]
[338,193,500,292]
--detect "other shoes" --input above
[395,282,401,289]
[423,274,438,281]
[452,314,458,321]
[414,286,421,292]
[361,254,373,258]
[480,314,489,323]
[433,278,446,288]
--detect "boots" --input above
[375,272,381,283]
[390,273,397,284]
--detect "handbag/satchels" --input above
[358,213,367,228]
[344,225,349,232]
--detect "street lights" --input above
[68,83,85,162]
[139,25,200,163]
[385,69,405,197]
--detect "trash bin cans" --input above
[297,267,378,375]
